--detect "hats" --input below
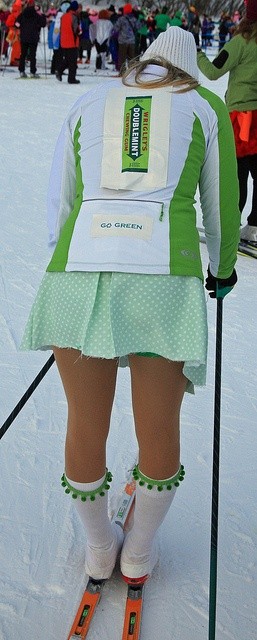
[189,6,195,13]
[138,26,199,82]
[109,4,115,13]
[124,4,132,15]
[61,2,70,12]
[70,1,78,10]
[27,0,35,7]
[98,10,109,20]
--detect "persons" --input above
[143,1,187,37]
[115,4,142,77]
[216,12,228,50]
[76,2,93,65]
[196,0,256,240]
[200,11,210,49]
[47,1,69,76]
[14,0,46,79]
[54,0,83,84]
[86,9,115,75]
[23,24,241,593]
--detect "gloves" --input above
[205,263,237,298]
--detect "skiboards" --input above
[196,227,257,258]
[65,449,148,640]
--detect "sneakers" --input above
[86,59,90,64]
[84,517,124,580]
[30,73,41,78]
[77,58,83,64]
[20,71,27,77]
[240,225,257,242]
[56,73,62,82]
[120,527,158,579]
[68,80,80,83]
